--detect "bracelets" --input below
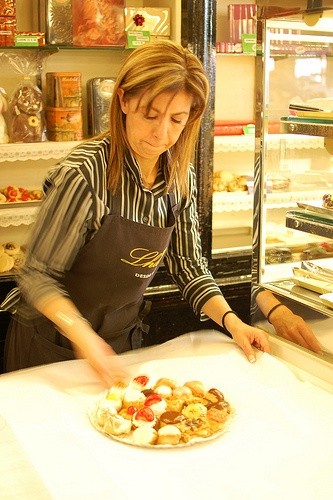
[267,303,283,323]
[222,311,238,334]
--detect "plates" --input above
[0,200,44,209]
[90,390,231,448]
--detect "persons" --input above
[251,285,322,355]
[0,40,271,388]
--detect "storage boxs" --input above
[0,0,170,143]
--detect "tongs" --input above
[292,260,332,283]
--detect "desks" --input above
[0,329,333,500]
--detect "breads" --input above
[212,170,251,192]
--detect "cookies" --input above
[8,87,43,143]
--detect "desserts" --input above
[0,186,43,203]
[0,241,25,273]
[97,373,232,444]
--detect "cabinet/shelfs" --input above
[250,0,333,341]
[0,0,333,300]
